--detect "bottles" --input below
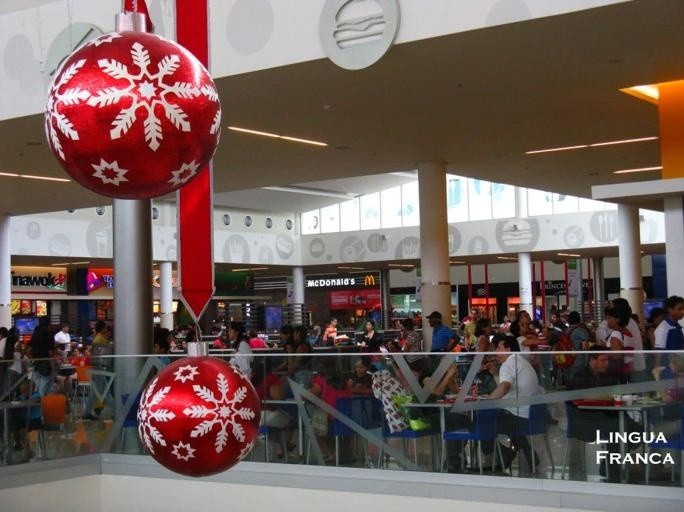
[614,394,622,408]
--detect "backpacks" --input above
[554,325,581,365]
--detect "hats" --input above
[427,311,442,319]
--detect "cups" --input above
[624,395,633,407]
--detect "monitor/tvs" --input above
[535,306,541,318]
[14,319,40,335]
[263,305,284,328]
[369,310,380,327]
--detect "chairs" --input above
[17,330,683,486]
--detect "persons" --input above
[1,296,684,478]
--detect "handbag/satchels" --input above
[310,374,329,438]
[393,396,431,430]
